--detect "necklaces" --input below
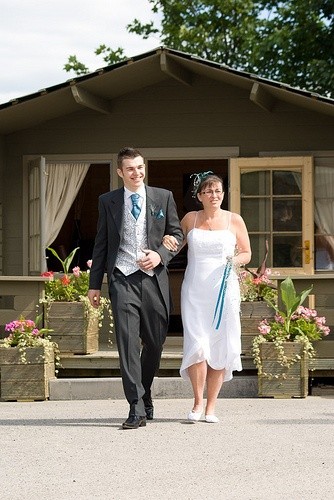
[203,210,221,231]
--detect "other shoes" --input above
[188,408,203,420]
[205,413,219,423]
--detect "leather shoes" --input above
[145,407,153,420]
[123,416,146,429]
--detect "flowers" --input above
[35,245,115,350]
[250,274,330,381]
[237,238,278,309]
[0,311,65,379]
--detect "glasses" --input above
[200,189,223,196]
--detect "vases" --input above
[257,341,309,400]
[241,301,277,356]
[49,302,100,354]
[0,346,55,402]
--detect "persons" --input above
[88,147,184,429]
[162,171,252,424]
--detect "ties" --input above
[130,194,141,220]
